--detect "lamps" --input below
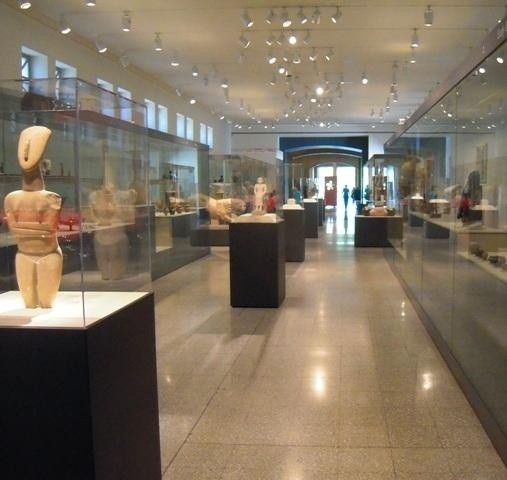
[210,67,399,130]
[392,9,433,73]
[19,0,209,108]
[235,6,343,69]
[404,53,505,128]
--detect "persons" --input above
[86,136,139,280]
[253,176,267,213]
[364,185,370,200]
[342,185,349,206]
[342,209,348,233]
[3,125,68,310]
[352,186,357,203]
